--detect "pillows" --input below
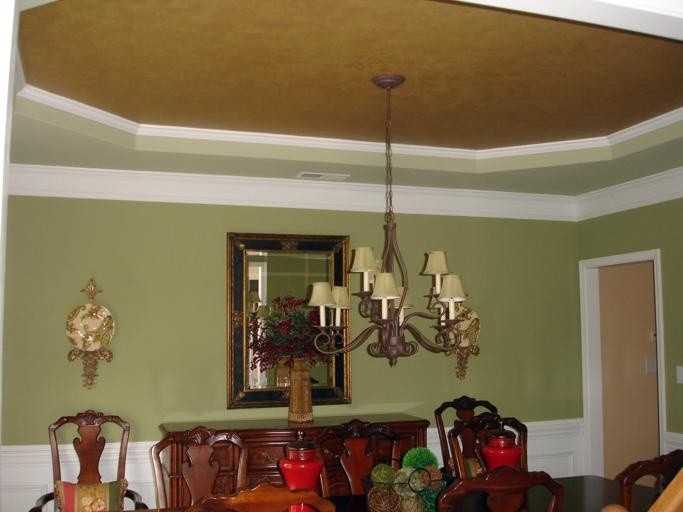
[55,479,126,511]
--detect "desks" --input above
[128,476,659,510]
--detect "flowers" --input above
[250,294,337,372]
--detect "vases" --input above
[287,356,314,423]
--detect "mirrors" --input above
[226,232,352,410]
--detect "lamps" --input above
[309,72,480,368]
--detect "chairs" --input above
[30,411,147,511]
[435,395,497,465]
[149,426,248,508]
[316,419,403,496]
[438,467,563,511]
[616,449,682,510]
[447,412,527,479]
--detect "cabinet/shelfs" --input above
[159,413,431,508]
